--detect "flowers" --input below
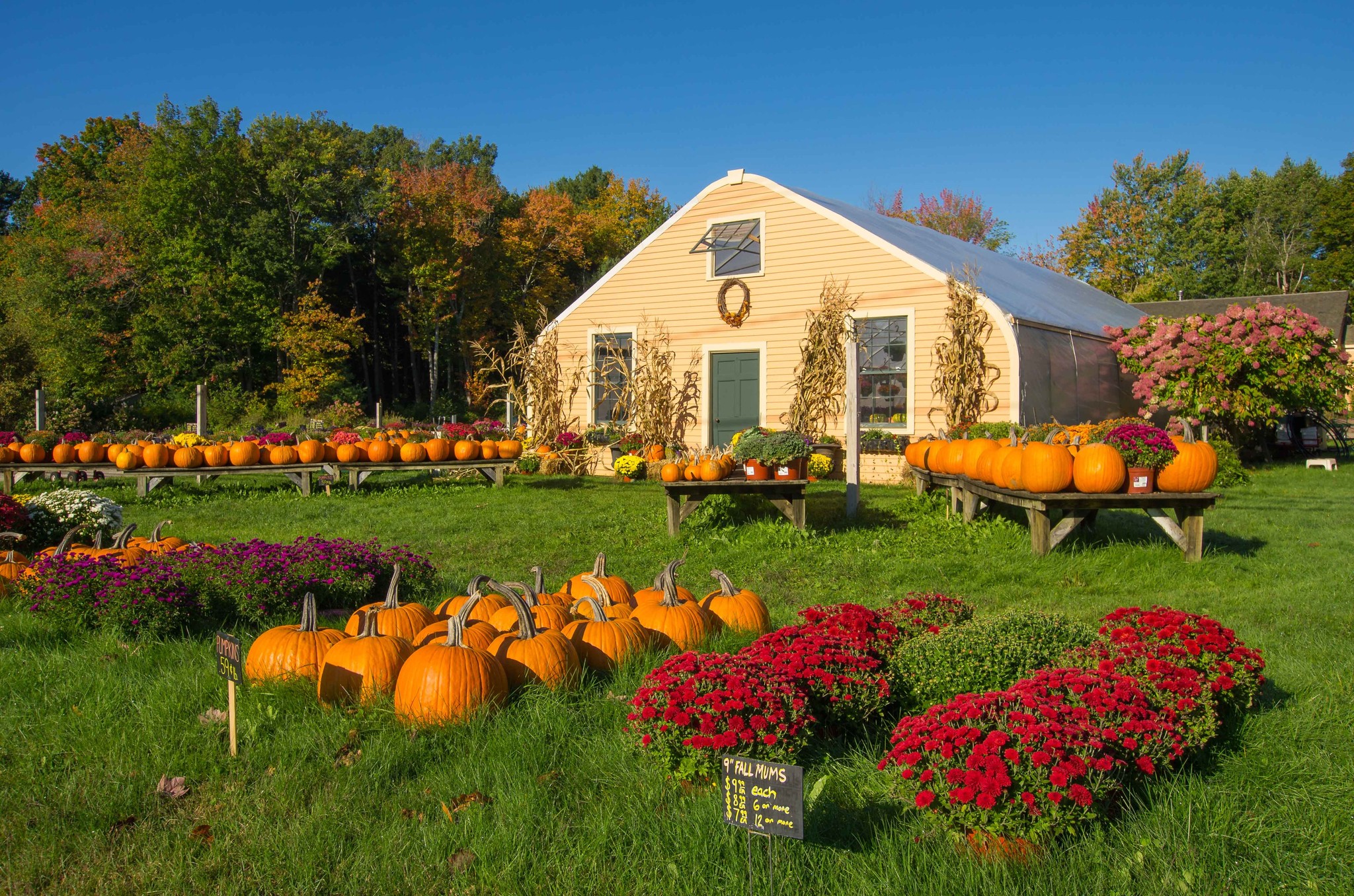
[860,364,907,396]
[0,414,1267,832]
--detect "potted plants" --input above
[811,434,842,458]
[883,340,907,362]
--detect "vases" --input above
[624,476,637,482]
[883,393,894,402]
[861,438,879,450]
[878,379,889,386]
[675,451,681,457]
[521,471,531,475]
[562,450,577,460]
[628,450,641,457]
[743,457,772,480]
[773,457,800,480]
[800,458,808,479]
[1122,467,1156,494]
[881,439,894,449]
[901,380,907,389]
[44,452,51,462]
[808,476,823,482]
[963,828,1047,867]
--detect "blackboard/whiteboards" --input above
[722,753,804,840]
[216,630,243,684]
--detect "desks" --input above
[327,457,519,494]
[116,463,329,498]
[660,465,810,540]
[909,465,962,513]
[954,472,1224,563]
[0,461,117,495]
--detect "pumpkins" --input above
[645,442,736,481]
[1153,418,1219,493]
[102,426,522,469]
[247,551,771,728]
[53,438,75,463]
[6,437,25,462]
[534,440,558,459]
[512,422,526,442]
[78,436,105,463]
[19,440,46,463]
[0,444,14,463]
[72,439,88,463]
[905,427,1127,493]
[0,520,218,599]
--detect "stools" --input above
[1306,458,1338,471]
